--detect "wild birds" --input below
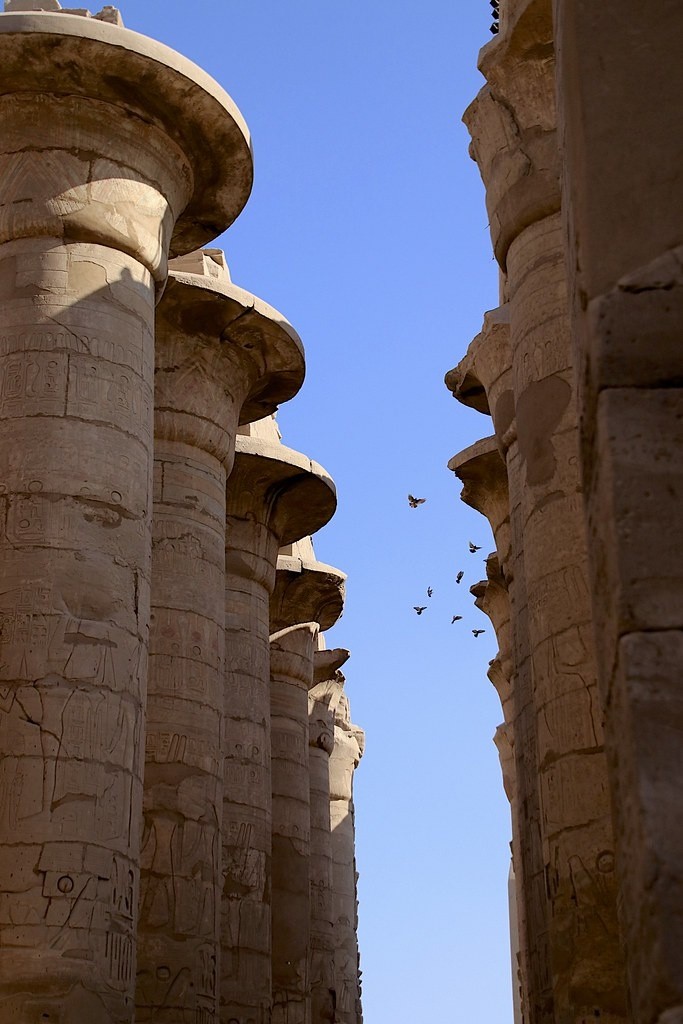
[412,606,428,616]
[455,570,465,584]
[471,629,486,638]
[450,614,463,625]
[468,541,483,554]
[426,585,434,598]
[407,492,427,510]
